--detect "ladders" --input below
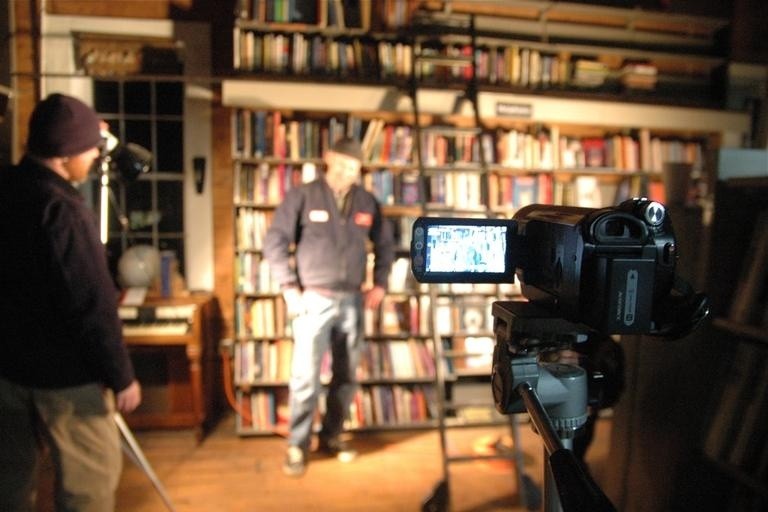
[409,12,542,512]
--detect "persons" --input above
[261,133,397,481]
[0,90,144,512]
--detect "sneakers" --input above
[319,435,356,461]
[285,446,312,475]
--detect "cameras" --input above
[409,196,711,357]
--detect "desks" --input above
[113,288,220,430]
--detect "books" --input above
[228,108,553,436]
[229,1,658,95]
[554,130,713,210]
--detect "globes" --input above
[117,245,161,288]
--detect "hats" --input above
[332,138,365,163]
[27,94,100,157]
